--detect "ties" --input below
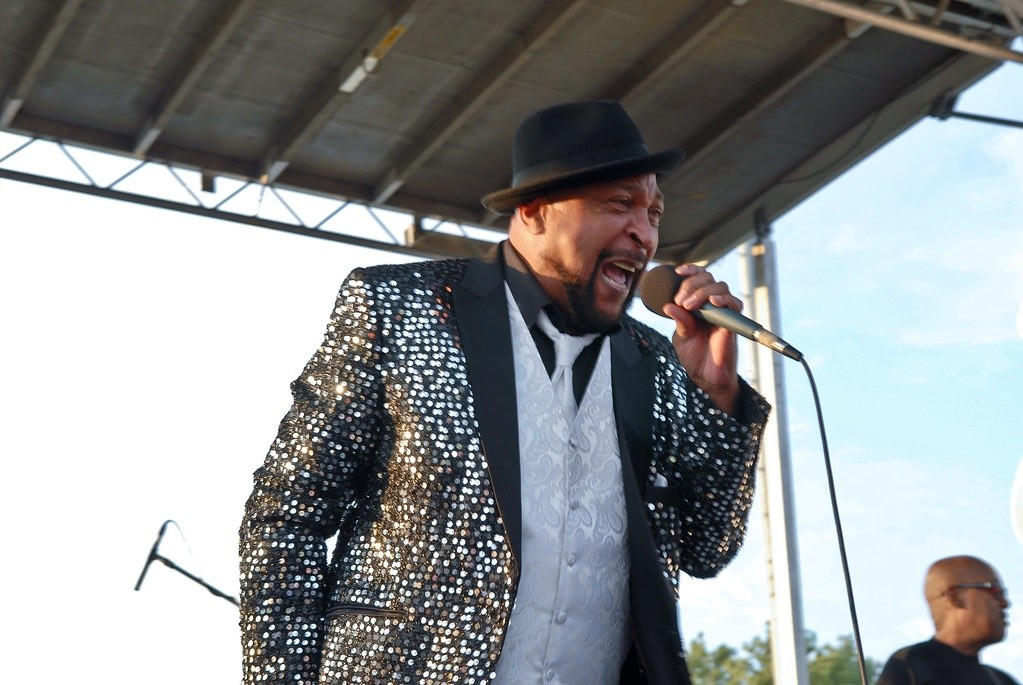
[535,308,601,429]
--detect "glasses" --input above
[942,581,1008,599]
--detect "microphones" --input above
[639,265,802,361]
[133,522,167,590]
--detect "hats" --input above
[482,101,687,215]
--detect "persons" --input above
[238,100,771,685]
[874,556,1023,685]
